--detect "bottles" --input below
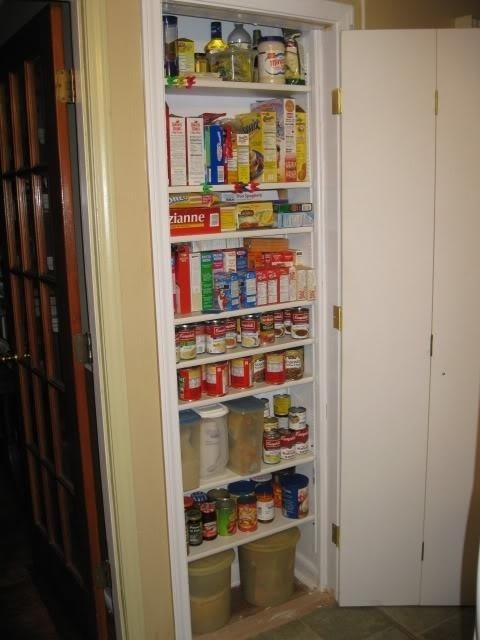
[227,23,252,48]
[164,15,178,77]
[204,22,226,52]
[252,28,263,82]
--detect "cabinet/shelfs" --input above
[72,1,480,640]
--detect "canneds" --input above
[175,307,309,402]
[262,394,309,464]
[257,36,286,84]
[194,53,210,73]
[183,474,273,556]
